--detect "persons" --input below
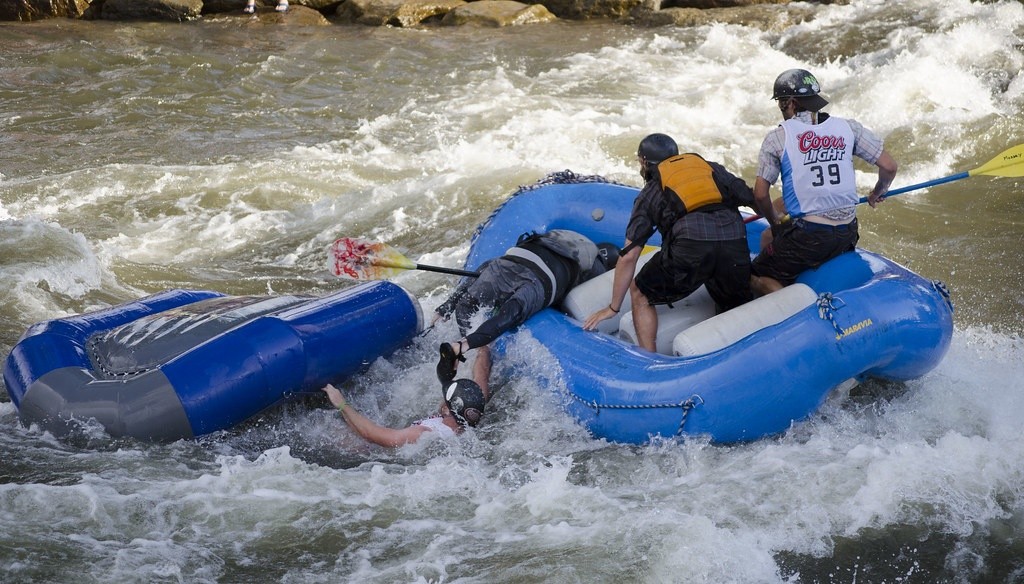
[322,347,491,450]
[750,68,898,296]
[432,229,622,389]
[582,134,765,352]
[242,0,289,15]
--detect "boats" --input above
[4,279,423,451]
[453,171,953,451]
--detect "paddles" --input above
[778,143,1024,224]
[741,196,787,225]
[327,236,481,282]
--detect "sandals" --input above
[437,341,467,386]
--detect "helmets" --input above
[638,133,678,165]
[442,379,485,429]
[769,68,821,100]
[595,242,621,272]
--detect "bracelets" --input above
[609,304,620,313]
[339,402,350,411]
